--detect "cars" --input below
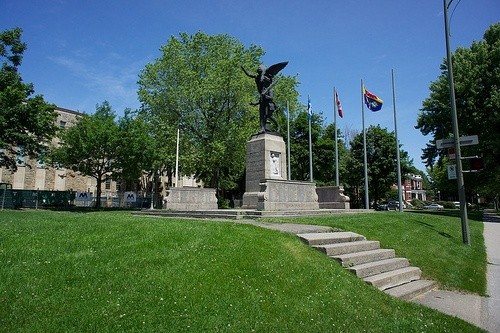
[377,201,399,211]
[396,202,406,209]
[453,202,471,208]
[423,204,443,210]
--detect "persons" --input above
[241,62,288,133]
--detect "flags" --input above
[283,106,288,118]
[307,98,313,119]
[335,92,344,118]
[363,87,383,112]
